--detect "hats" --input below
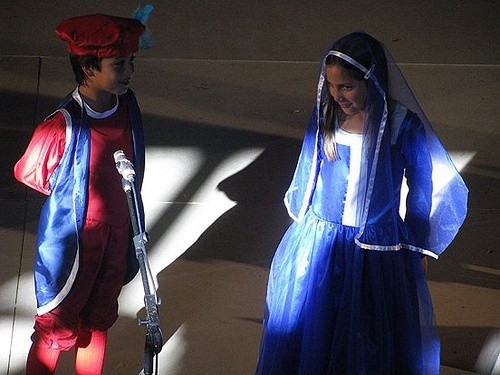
[53,13,146,57]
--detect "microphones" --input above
[113,150,135,182]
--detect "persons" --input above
[14,14,145,375]
[255,33,433,375]
